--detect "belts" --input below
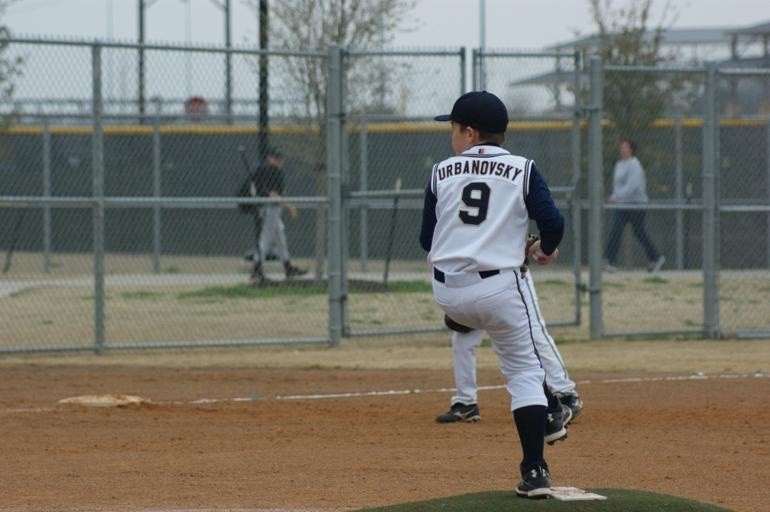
[434,266,499,283]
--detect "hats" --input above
[434,91,508,132]
[266,145,285,158]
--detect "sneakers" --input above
[648,256,665,273]
[437,404,480,422]
[544,396,573,445]
[251,273,276,287]
[287,266,307,276]
[601,261,617,272]
[516,466,551,497]
[560,395,584,415]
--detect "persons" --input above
[433,264,584,425]
[247,144,309,282]
[419,88,573,498]
[600,137,667,274]
